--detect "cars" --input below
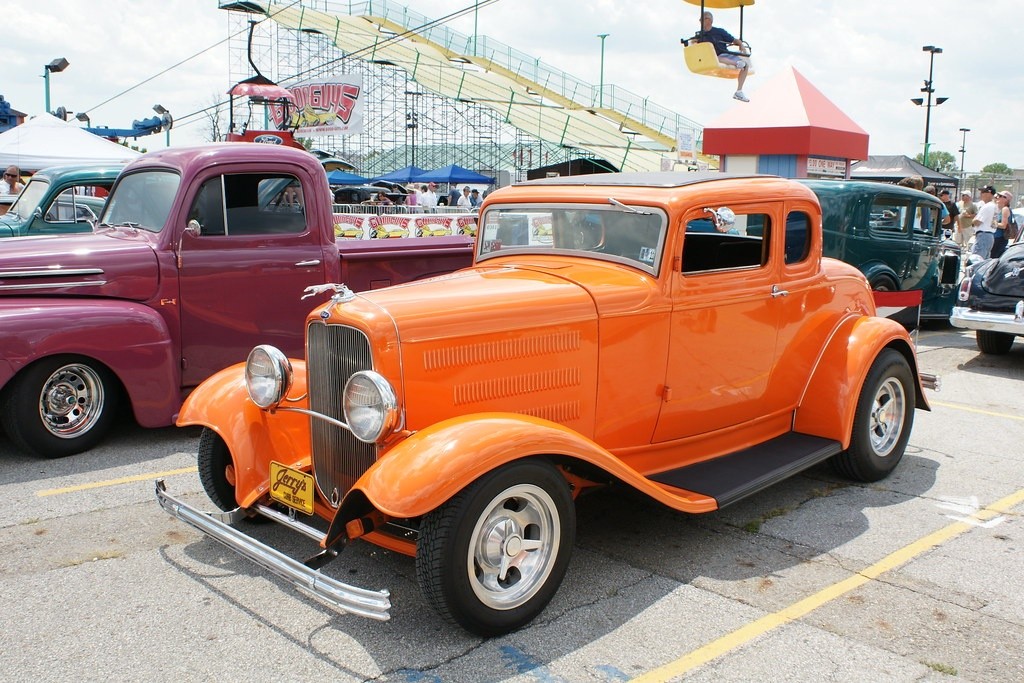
[0,166,121,237]
[788,179,984,332]
[156,172,939,638]
[950,222,1024,356]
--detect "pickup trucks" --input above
[0,142,476,459]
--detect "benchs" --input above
[622,232,763,272]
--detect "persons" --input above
[953,191,978,246]
[990,191,1012,258]
[447,183,462,206]
[923,186,950,225]
[287,186,303,206]
[939,189,963,269]
[456,186,479,213]
[896,175,924,228]
[370,190,393,214]
[691,12,749,102]
[406,182,437,213]
[392,183,403,205]
[0,164,25,195]
[972,186,999,260]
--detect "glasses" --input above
[5,173,17,178]
[432,186,435,187]
[998,194,1007,198]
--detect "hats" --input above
[977,184,996,196]
[961,189,972,198]
[429,182,438,186]
[997,191,1013,201]
[938,190,950,195]
[472,189,480,193]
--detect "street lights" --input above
[153,104,170,147]
[404,91,422,165]
[911,46,949,168]
[597,34,608,107]
[77,112,90,129]
[959,127,971,200]
[39,57,69,113]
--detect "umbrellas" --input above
[0,112,143,169]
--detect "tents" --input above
[408,164,496,195]
[371,165,428,182]
[325,169,372,185]
[850,155,959,201]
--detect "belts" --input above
[976,230,992,233]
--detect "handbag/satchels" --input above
[1000,206,1018,239]
